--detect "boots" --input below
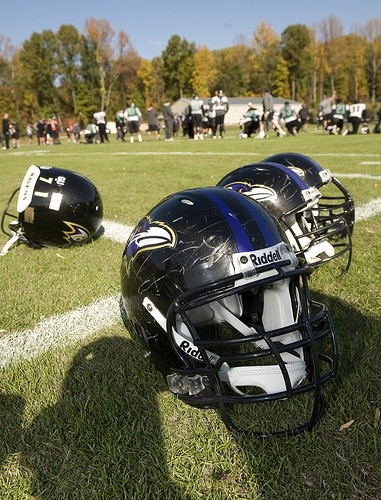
[138,134,142,142]
[130,136,135,143]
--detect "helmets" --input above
[217,163,335,264]
[261,152,332,217]
[121,187,307,411]
[8,164,103,245]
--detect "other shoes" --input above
[194,133,222,140]
[342,129,348,136]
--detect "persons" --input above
[162,89,229,141]
[92,109,109,144]
[255,87,286,139]
[0,113,20,149]
[147,104,160,141]
[34,117,61,145]
[316,91,381,136]
[26,122,33,145]
[64,119,100,145]
[238,101,312,138]
[114,101,142,143]
